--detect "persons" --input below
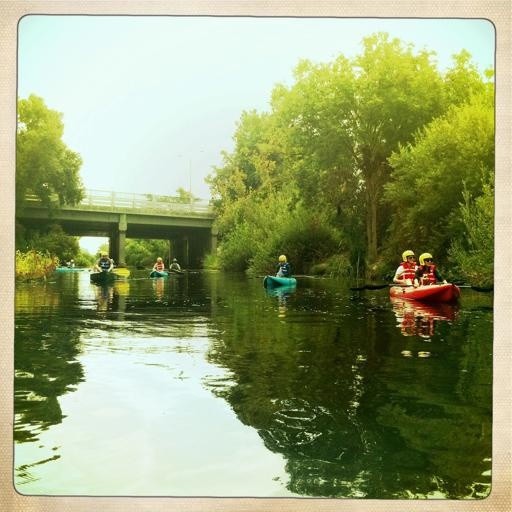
[412,253,447,288]
[109,255,115,266]
[95,252,113,273]
[275,255,292,278]
[68,259,75,267]
[393,249,419,286]
[169,258,180,272]
[152,257,165,272]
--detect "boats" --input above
[389,283,461,303]
[262,275,296,288]
[150,269,167,278]
[90,266,129,283]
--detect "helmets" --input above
[157,258,162,262]
[279,255,286,262]
[419,253,433,265]
[402,250,414,262]
[102,253,108,256]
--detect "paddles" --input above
[248,275,322,281]
[110,268,130,278]
[136,265,175,274]
[350,279,464,292]
[365,282,494,292]
